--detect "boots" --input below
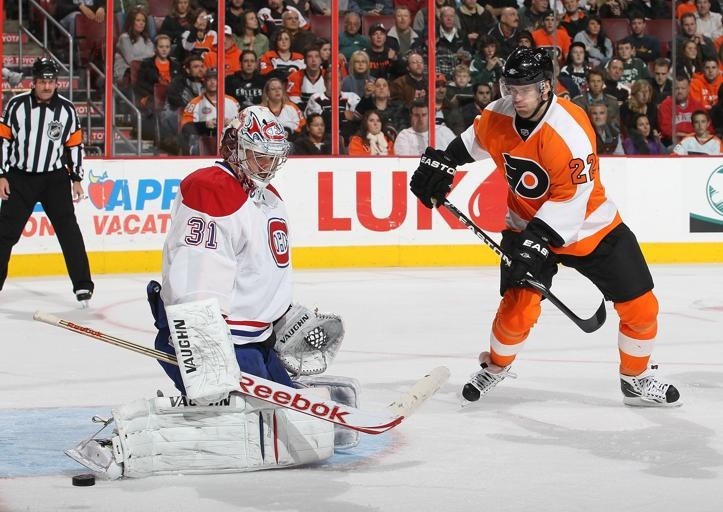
[619,366,679,404]
[461,350,513,401]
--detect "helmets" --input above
[498,46,553,102]
[217,106,291,203]
[30,58,58,81]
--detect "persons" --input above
[0,58,97,301]
[2,67,25,87]
[25,0,723,156]
[62,105,359,481]
[409,47,680,408]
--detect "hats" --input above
[369,23,384,35]
[203,66,217,79]
[223,26,232,38]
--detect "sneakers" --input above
[73,288,93,301]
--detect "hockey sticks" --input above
[31,311,450,434]
[441,198,607,332]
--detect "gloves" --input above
[409,147,456,209]
[504,230,552,285]
